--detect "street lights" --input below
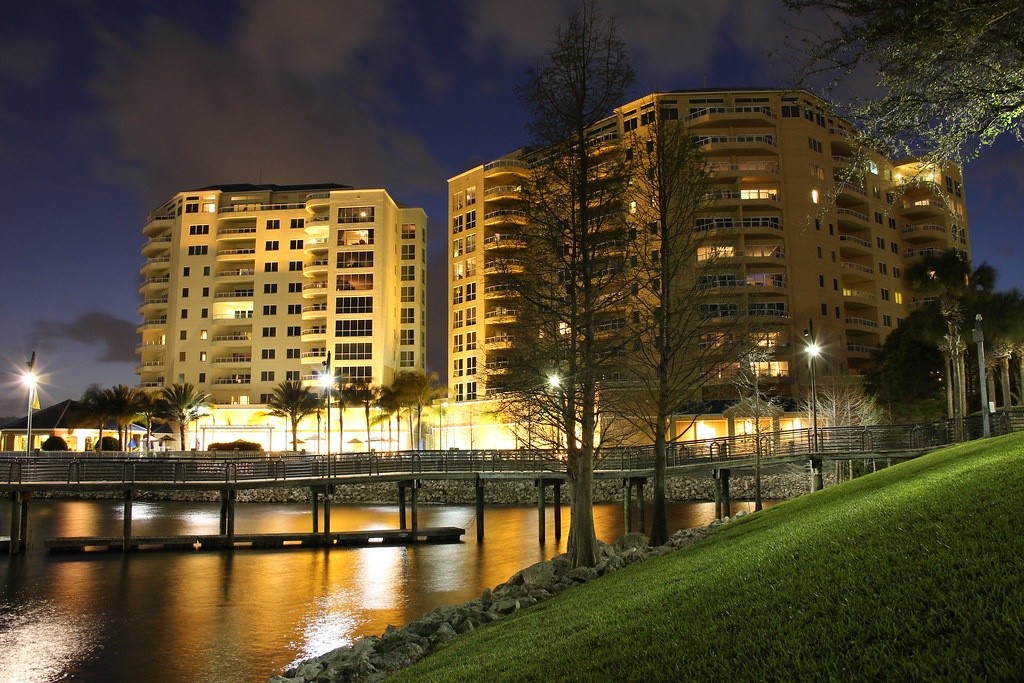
[439,402,447,452]
[321,350,330,477]
[22,351,37,460]
[808,318,818,452]
